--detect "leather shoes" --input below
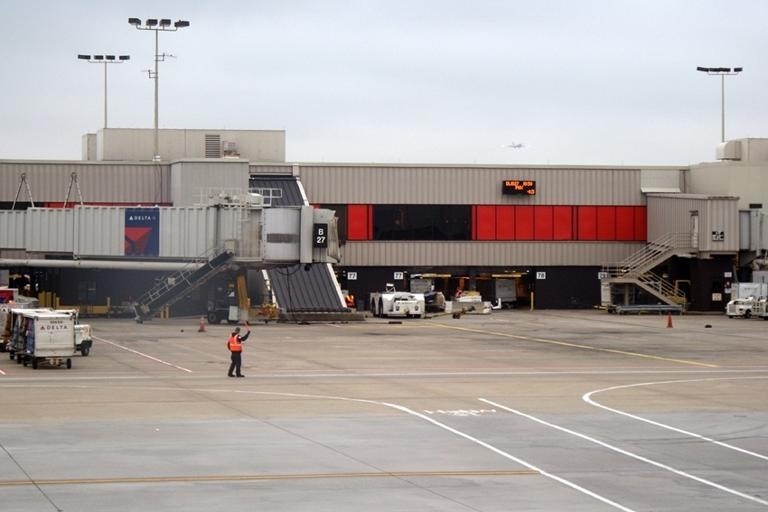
[228,374,245,377]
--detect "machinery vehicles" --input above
[370,283,425,318]
[726,296,768,319]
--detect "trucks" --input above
[9,308,92,369]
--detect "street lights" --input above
[129,18,190,161]
[697,67,743,142]
[78,55,130,128]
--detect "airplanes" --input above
[510,141,522,148]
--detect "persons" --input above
[226,326,251,377]
[344,291,355,308]
[455,286,462,297]
